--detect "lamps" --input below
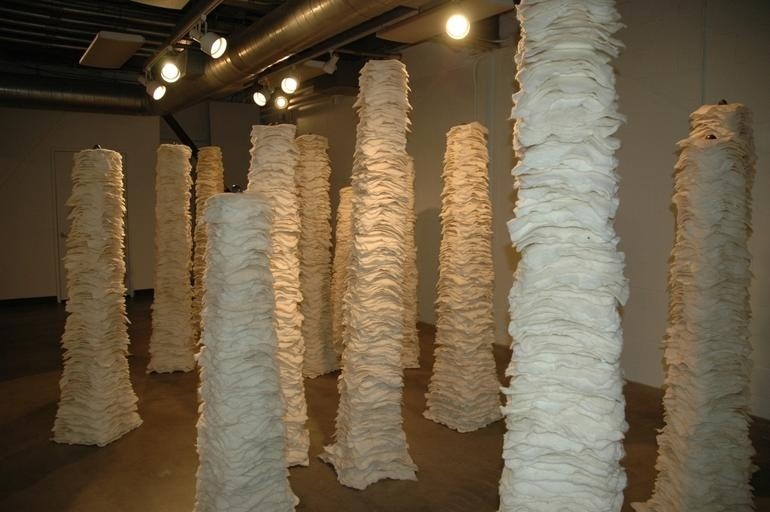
[138,16,228,104]
